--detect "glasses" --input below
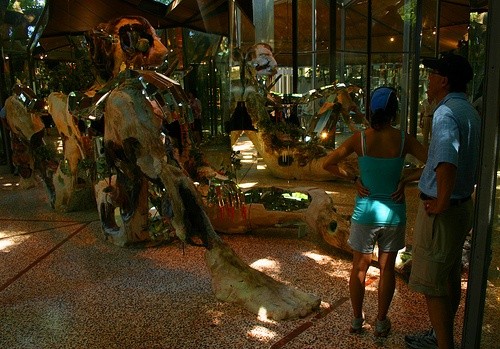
[429,70,446,78]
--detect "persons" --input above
[322,87,427,338]
[10,83,38,171]
[190,90,202,131]
[404,54,481,349]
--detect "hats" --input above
[422,53,474,83]
[370,87,397,117]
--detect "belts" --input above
[421,192,472,204]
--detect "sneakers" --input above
[376,316,391,332]
[405,327,438,349]
[351,311,365,329]
